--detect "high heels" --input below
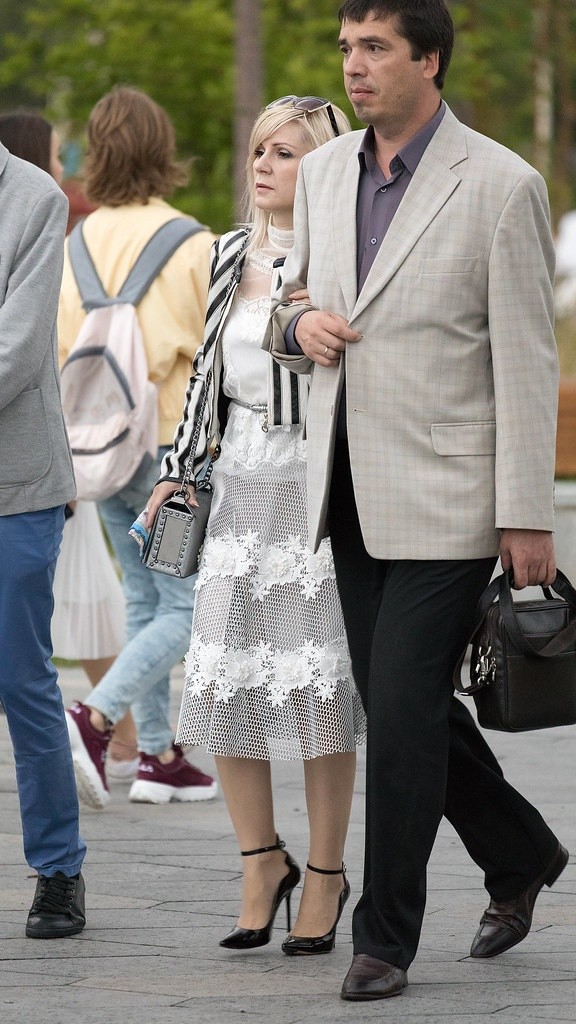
[219,833,301,949]
[281,861,350,953]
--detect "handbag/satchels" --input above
[452,565,576,732]
[141,491,213,579]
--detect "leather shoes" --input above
[340,954,408,998]
[470,841,570,958]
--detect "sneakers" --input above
[63,700,115,810]
[25,871,87,938]
[127,742,218,805]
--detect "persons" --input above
[0,141,87,937]
[0,114,65,186]
[260,0,570,999]
[144,94,367,953]
[55,86,219,809]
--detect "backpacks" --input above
[59,216,208,502]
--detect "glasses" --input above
[266,95,339,137]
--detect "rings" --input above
[324,347,329,358]
